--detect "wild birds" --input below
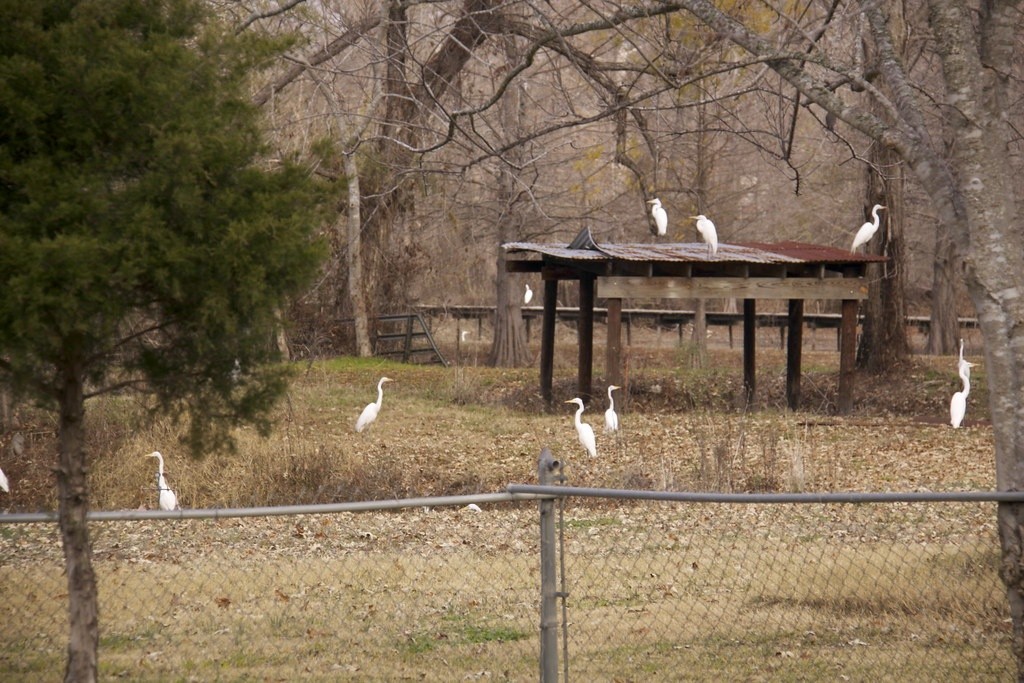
[144,451,182,525]
[461,330,470,342]
[645,197,668,237]
[688,214,717,259]
[849,203,887,256]
[355,376,394,434]
[564,397,598,463]
[0,468,10,495]
[947,338,981,430]
[602,384,623,435]
[523,282,533,308]
